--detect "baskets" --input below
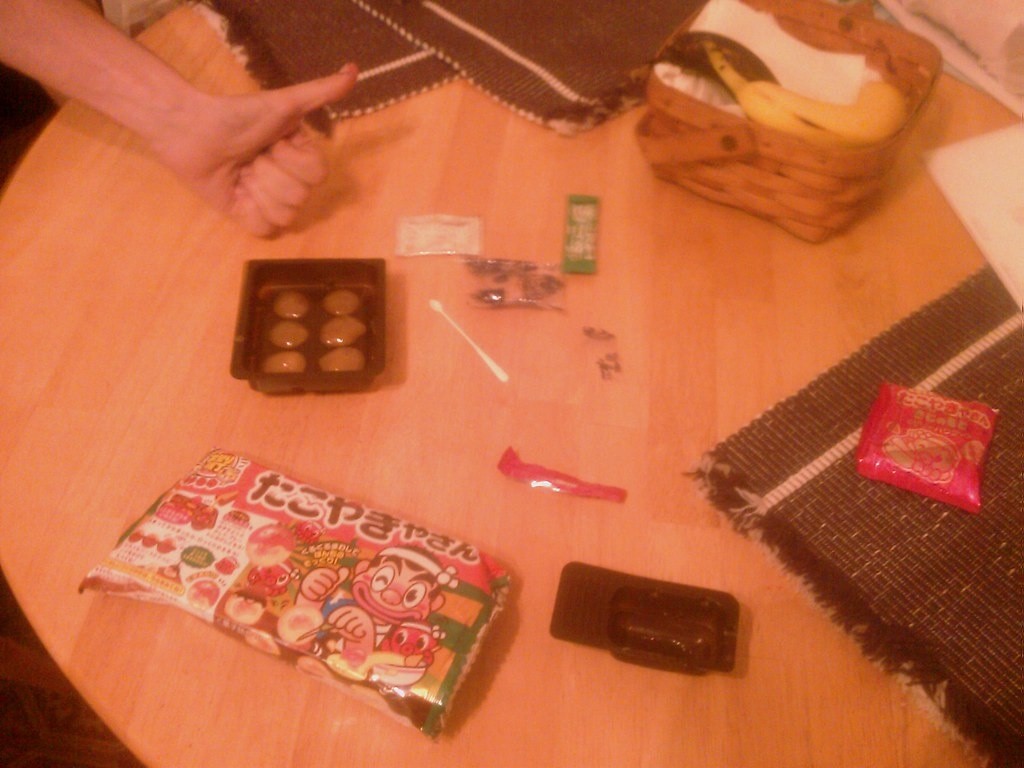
[635,0,943,243]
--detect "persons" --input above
[0,1,357,238]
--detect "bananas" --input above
[736,77,907,146]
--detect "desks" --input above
[0,0,1023,768]
